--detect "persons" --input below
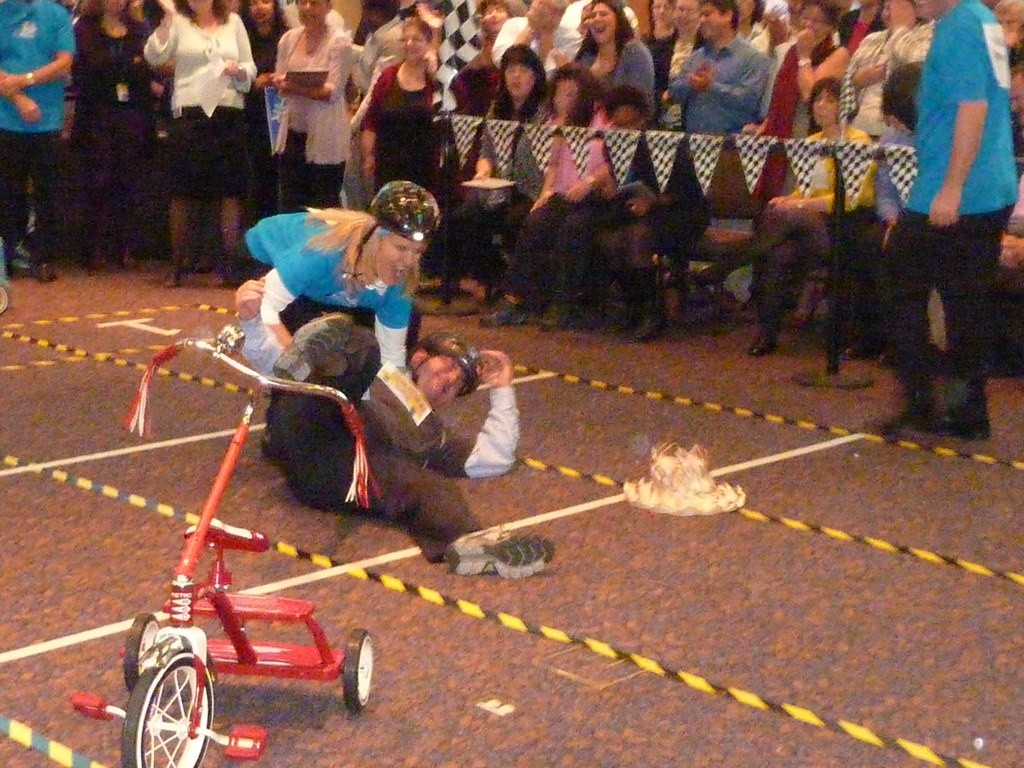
[3,1,1024,429]
[231,179,559,577]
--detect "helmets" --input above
[407,331,482,398]
[369,179,442,241]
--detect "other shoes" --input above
[419,259,665,344]
[933,396,991,440]
[442,525,555,580]
[272,313,354,382]
[4,230,270,293]
[688,263,724,287]
[749,330,777,356]
[877,400,941,443]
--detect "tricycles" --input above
[70,324,375,767]
